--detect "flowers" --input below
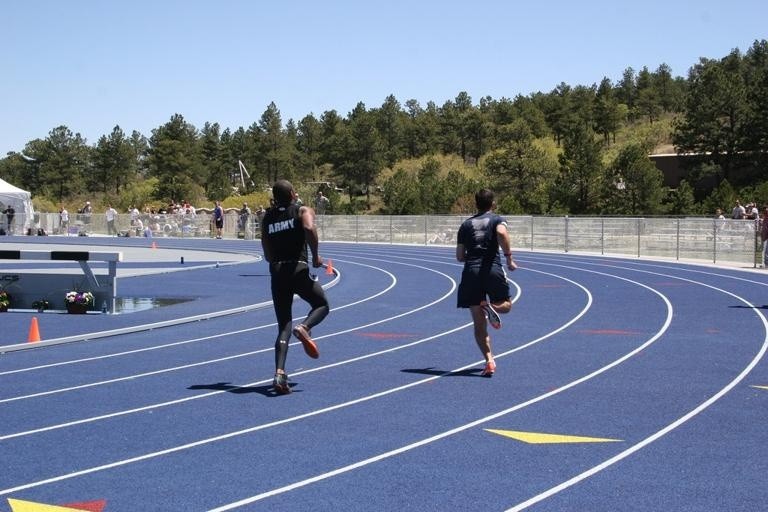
[1,287,11,311]
[65,290,95,308]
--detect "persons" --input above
[270,198,275,208]
[59,205,71,236]
[294,193,304,207]
[75,198,268,241]
[2,205,15,234]
[262,181,330,395]
[314,191,330,215]
[451,187,519,376]
[713,198,767,270]
[32,210,42,231]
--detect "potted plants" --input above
[67,304,89,316]
[31,297,48,313]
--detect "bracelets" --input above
[504,253,513,256]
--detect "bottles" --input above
[181,257,184,264]
[215,260,219,269]
[102,300,108,318]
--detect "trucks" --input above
[301,180,343,196]
[261,183,272,193]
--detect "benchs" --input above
[511,215,762,253]
[317,214,462,243]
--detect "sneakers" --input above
[482,359,496,378]
[273,373,293,394]
[481,300,503,330]
[293,324,319,359]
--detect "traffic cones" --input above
[322,258,335,276]
[26,316,41,343]
[151,241,157,250]
